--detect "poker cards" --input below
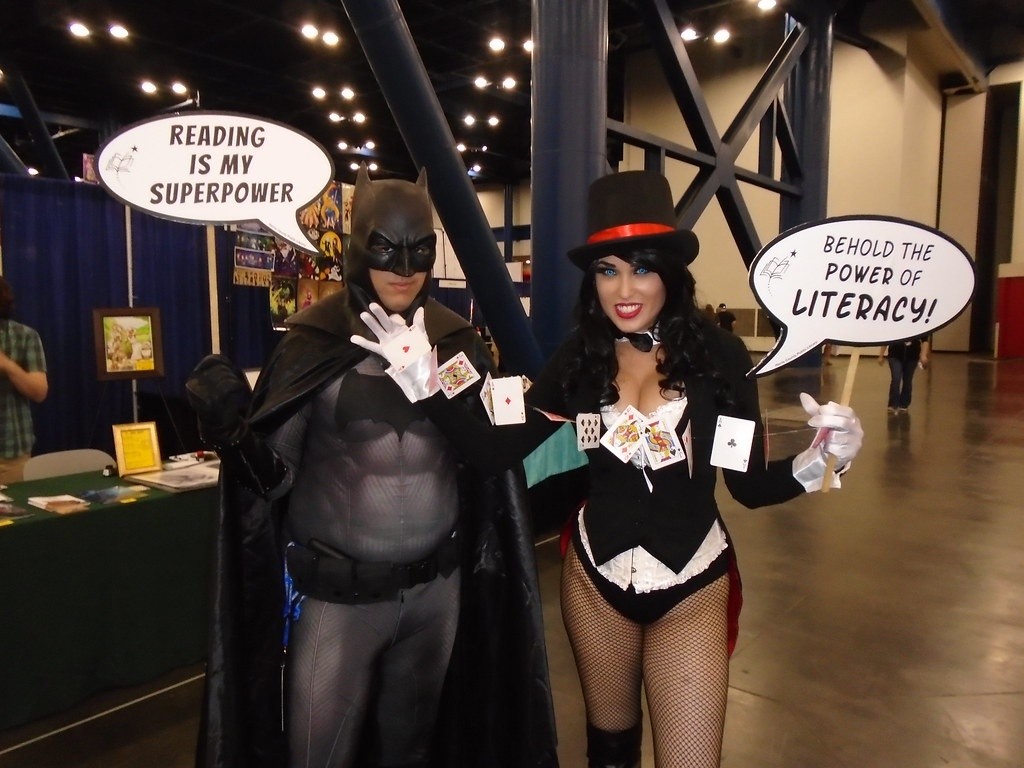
[382,324,433,373]
[635,413,686,472]
[577,414,600,451]
[436,351,483,400]
[480,372,527,426]
[600,405,649,464]
[535,408,576,421]
[710,415,755,473]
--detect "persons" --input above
[180,160,560,768]
[718,303,736,333]
[704,304,720,326]
[349,170,865,768]
[878,332,929,415]
[0,274,48,465]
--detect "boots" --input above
[586,710,643,768]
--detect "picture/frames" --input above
[111,421,164,478]
[92,307,165,382]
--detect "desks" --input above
[0,460,220,731]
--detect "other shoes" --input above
[887,407,908,415]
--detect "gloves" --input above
[350,302,432,372]
[186,354,250,446]
[799,393,863,471]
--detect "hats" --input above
[568,171,700,273]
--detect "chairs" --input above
[22,449,117,482]
